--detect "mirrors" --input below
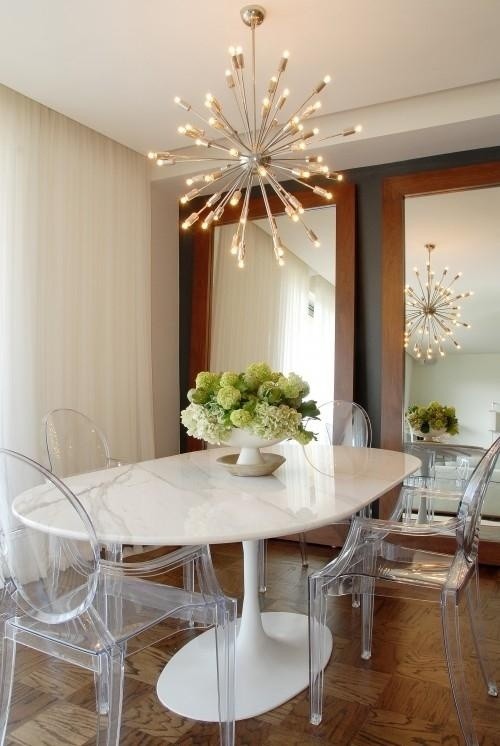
[184,185,356,448]
[380,163,499,567]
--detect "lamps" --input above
[404,243,474,359]
[147,6,365,268]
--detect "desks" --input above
[15,448,412,721]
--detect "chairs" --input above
[304,439,499,745]
[1,443,236,746]
[298,400,375,572]
[42,407,221,596]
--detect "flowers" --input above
[180,362,322,450]
[406,401,460,438]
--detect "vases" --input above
[415,431,446,445]
[220,431,288,464]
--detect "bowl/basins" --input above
[215,453,287,477]
